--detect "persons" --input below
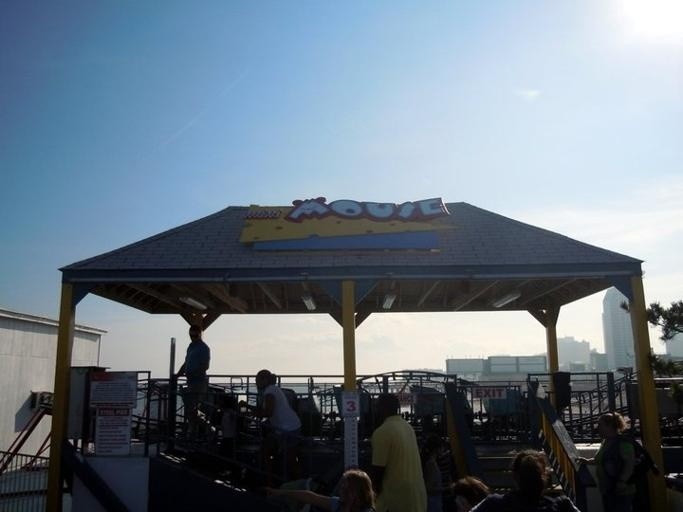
[446,476,489,512]
[238,369,302,442]
[468,449,559,512]
[176,324,210,412]
[572,410,637,512]
[261,467,376,512]
[625,435,660,512]
[420,434,456,512]
[442,381,482,476]
[218,393,241,460]
[368,393,428,512]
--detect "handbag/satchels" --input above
[618,434,654,485]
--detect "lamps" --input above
[493,290,520,308]
[179,296,207,310]
[301,295,315,311]
[383,291,396,310]
